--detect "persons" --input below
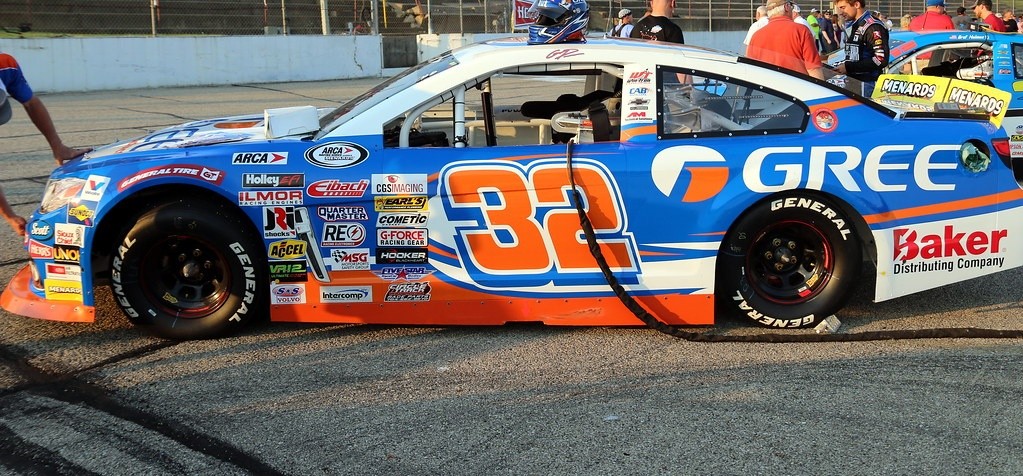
[610,0,691,91]
[0,53,93,237]
[743,0,1023,104]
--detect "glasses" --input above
[826,14,831,15]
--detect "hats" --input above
[957,7,966,15]
[792,4,801,12]
[0,79,12,127]
[825,10,830,14]
[971,0,992,9]
[811,8,820,14]
[926,0,947,7]
[872,10,880,15]
[766,0,795,11]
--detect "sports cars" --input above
[0,37,1023,329]
[820,26,1022,177]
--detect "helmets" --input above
[527,0,590,44]
